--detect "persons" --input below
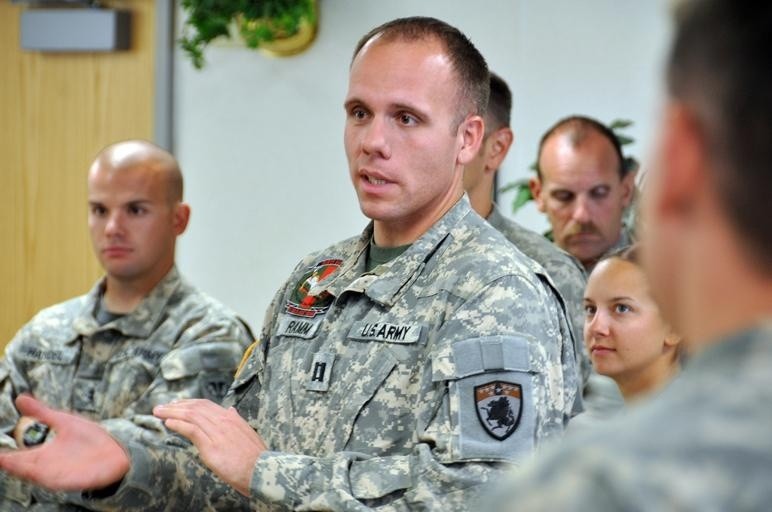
[0,138,254,512]
[462,72,593,395]
[527,116,636,270]
[585,245,691,403]
[464,0,772,512]
[0,16,586,512]
